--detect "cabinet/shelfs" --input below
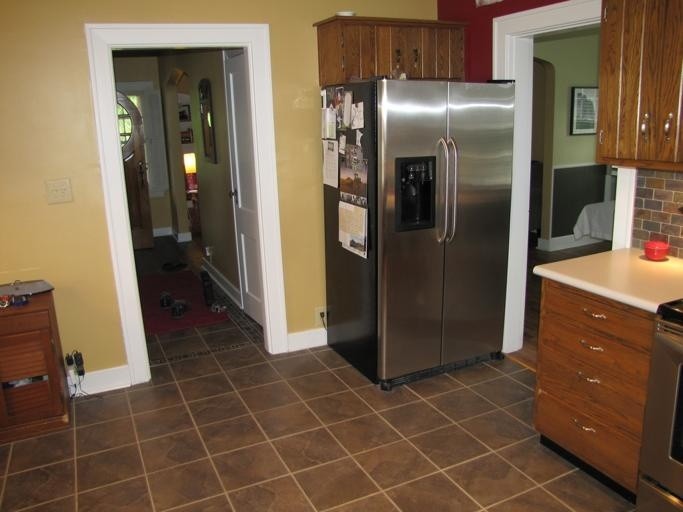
[0,279,70,448]
[533,275,655,501]
[313,13,469,84]
[596,1,681,172]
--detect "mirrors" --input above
[198,78,217,163]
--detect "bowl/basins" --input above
[644,241,669,261]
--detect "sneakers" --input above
[172,301,185,316]
[161,294,170,308]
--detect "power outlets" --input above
[45,177,73,205]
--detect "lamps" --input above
[183,152,197,189]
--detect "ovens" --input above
[631,297,682,512]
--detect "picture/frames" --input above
[567,87,597,134]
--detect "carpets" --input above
[138,269,229,336]
